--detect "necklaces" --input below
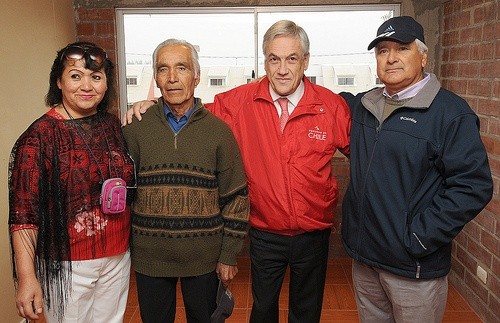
[68,113,120,158]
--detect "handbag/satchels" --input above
[100,177,127,214]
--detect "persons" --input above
[248,16,493,323]
[8,41,136,323]
[120,38,247,323]
[123,20,351,323]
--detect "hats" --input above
[367,15,425,51]
[209,279,234,323]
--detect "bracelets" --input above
[150,100,157,104]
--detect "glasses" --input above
[60,45,109,64]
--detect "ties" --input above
[277,98,290,133]
